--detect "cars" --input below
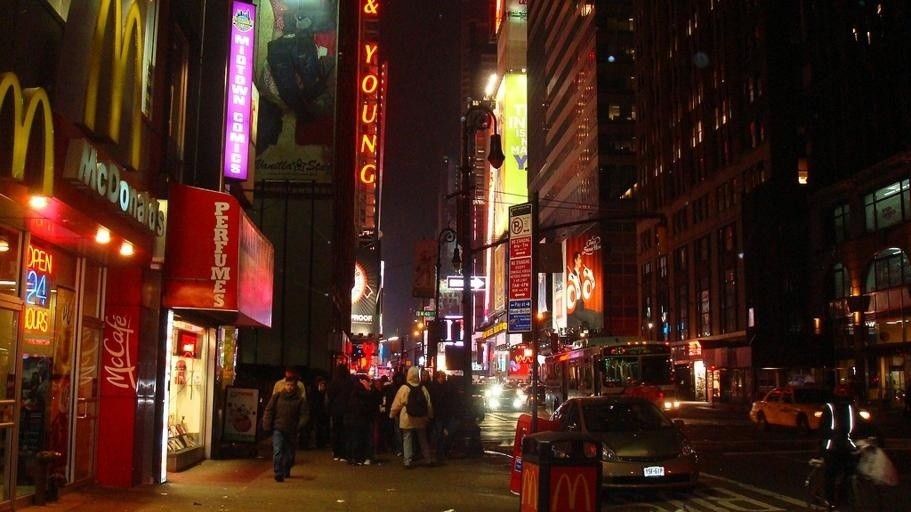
[445,371,546,412]
[547,394,700,497]
[749,383,874,437]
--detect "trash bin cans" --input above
[519,430,603,512]
[510,414,557,496]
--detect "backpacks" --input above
[405,383,427,416]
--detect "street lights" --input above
[432,227,462,382]
[447,104,505,455]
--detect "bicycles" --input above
[429,414,456,466]
[804,438,907,511]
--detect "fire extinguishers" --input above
[175,360,186,384]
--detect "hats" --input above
[316,376,327,384]
[834,385,848,396]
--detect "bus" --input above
[547,332,681,422]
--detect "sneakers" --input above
[824,497,837,507]
[274,473,284,481]
[404,458,412,466]
[425,455,439,464]
[334,455,348,462]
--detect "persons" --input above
[262,373,312,482]
[572,251,585,310]
[296,364,449,469]
[818,379,859,510]
[271,362,311,468]
